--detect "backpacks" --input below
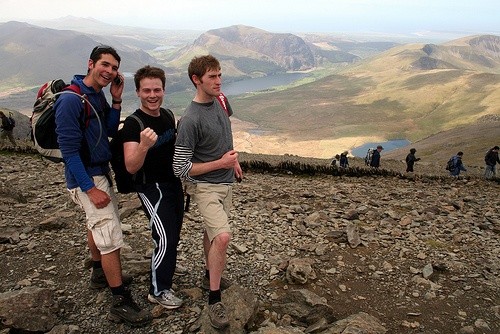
[6,116,15,128]
[446,156,461,172]
[363,148,374,166]
[30,78,106,164]
[109,108,177,194]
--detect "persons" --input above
[339,151,349,168]
[172,55,243,329]
[405,148,421,172]
[329,154,340,167]
[484,145,500,177]
[0,111,18,148]
[121,65,186,308]
[52,43,154,326]
[450,151,468,175]
[370,145,384,168]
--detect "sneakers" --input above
[202,274,231,290]
[206,301,231,330]
[148,290,184,310]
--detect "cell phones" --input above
[234,173,242,183]
[114,75,122,85]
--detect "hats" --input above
[376,146,385,150]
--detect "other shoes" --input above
[89,266,136,290]
[107,293,153,327]
